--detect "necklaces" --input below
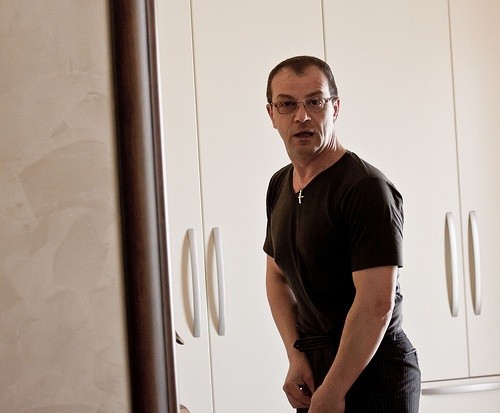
[295,146,343,205]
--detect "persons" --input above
[265,56,422,413]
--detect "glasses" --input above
[268,96,336,115]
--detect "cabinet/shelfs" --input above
[144,0,500,413]
